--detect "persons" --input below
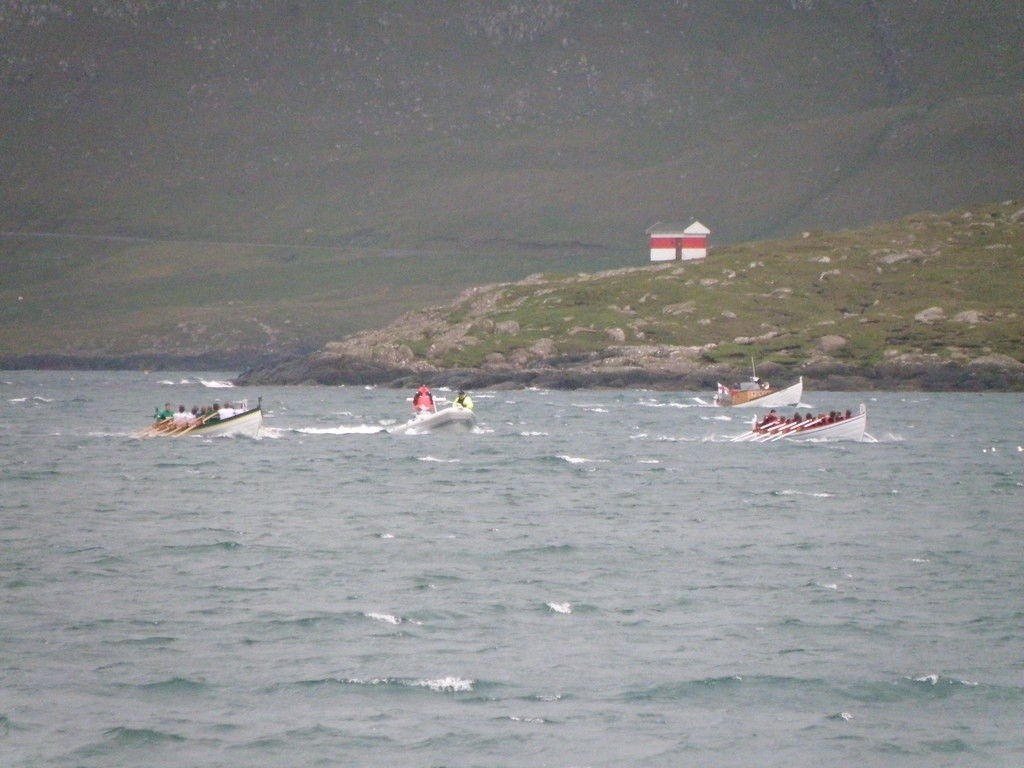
[452,392,473,411]
[412,383,433,415]
[756,410,853,434]
[172,401,248,429]
[161,403,174,419]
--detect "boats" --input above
[405,401,476,433]
[750,403,866,443]
[714,375,802,410]
[155,397,262,439]
